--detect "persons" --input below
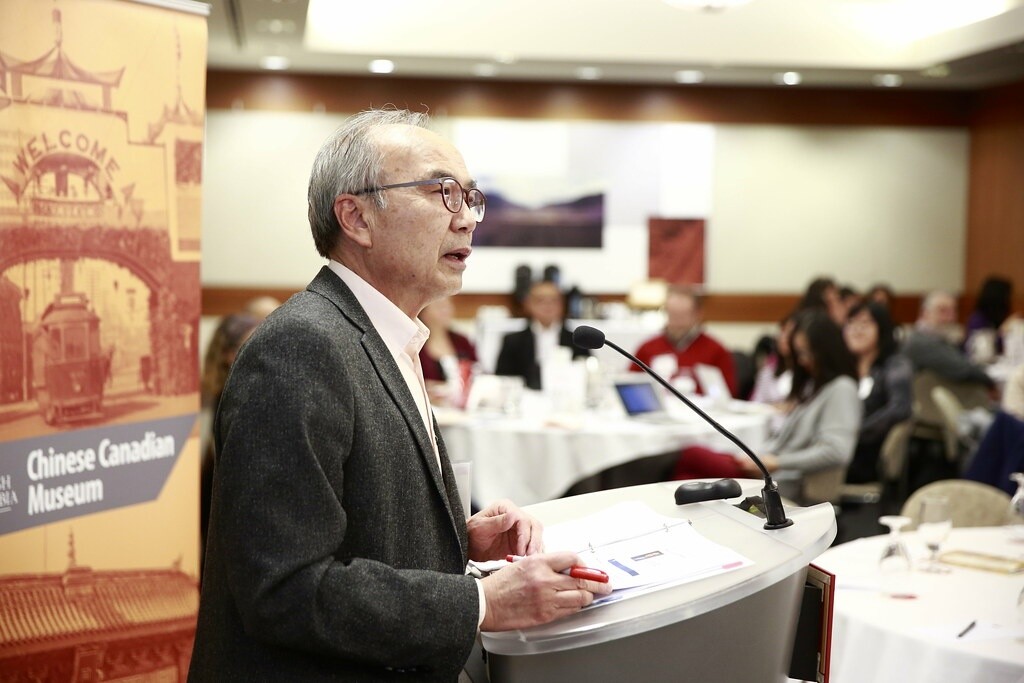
[495,265,593,397]
[246,297,281,322]
[200,311,262,414]
[741,276,1023,511]
[186,105,612,683]
[418,296,477,410]
[629,286,737,396]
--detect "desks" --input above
[429,388,769,514]
[810,527,1024,683]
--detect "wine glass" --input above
[1007,473,1024,545]
[875,516,915,598]
[918,495,951,574]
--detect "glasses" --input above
[348,178,486,223]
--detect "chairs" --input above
[812,368,1024,534]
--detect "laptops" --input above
[608,371,689,423]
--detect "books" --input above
[466,499,754,617]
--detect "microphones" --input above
[572,326,793,530]
